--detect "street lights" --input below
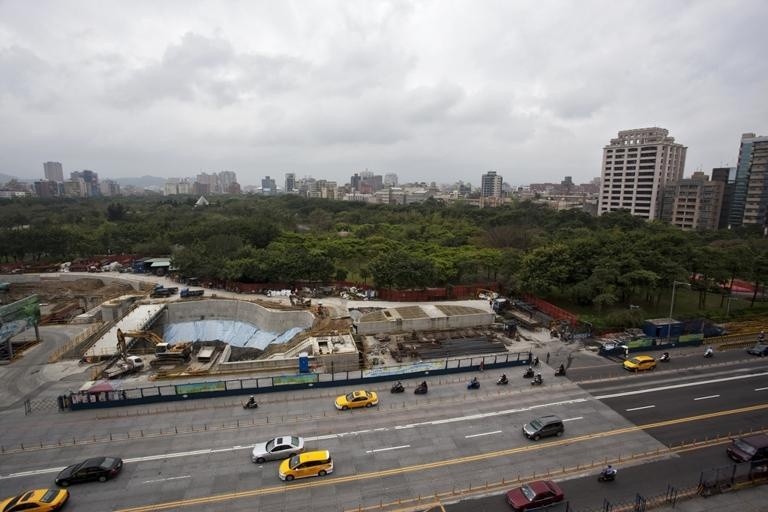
[666,281,692,342]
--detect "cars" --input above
[335,389,379,408]
[747,342,768,356]
[623,355,656,372]
[523,414,564,441]
[151,289,171,299]
[0,488,69,511]
[506,480,564,511]
[726,433,768,461]
[251,436,334,481]
[55,457,122,486]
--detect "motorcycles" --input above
[598,469,618,480]
[554,368,566,376]
[660,354,673,362]
[467,381,480,390]
[523,370,544,385]
[414,384,428,395]
[390,385,404,393]
[242,401,259,410]
[703,351,714,356]
[496,376,508,385]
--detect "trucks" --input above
[103,355,144,376]
[153,284,178,294]
[181,287,205,298]
[493,297,544,329]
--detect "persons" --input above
[603,465,613,480]
[559,364,565,373]
[546,352,550,363]
[470,377,477,386]
[246,395,254,407]
[394,380,402,390]
[419,381,427,391]
[500,373,506,382]
[537,374,541,384]
[526,367,533,375]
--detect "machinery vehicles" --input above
[114,327,192,367]
[288,293,312,308]
